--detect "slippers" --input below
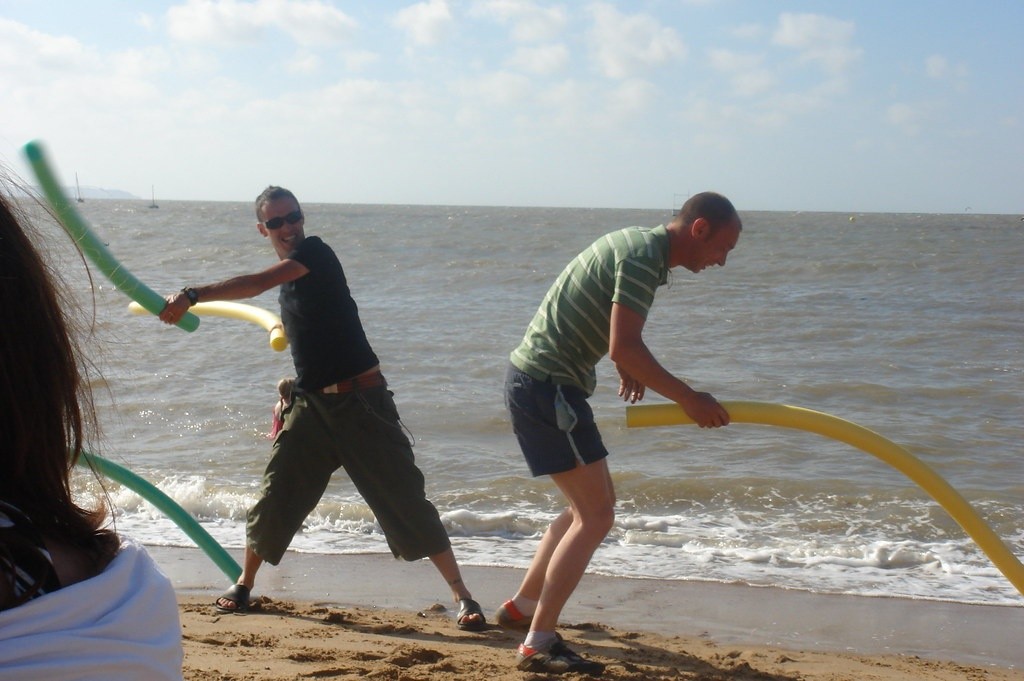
[456,599,485,630]
[215,584,250,610]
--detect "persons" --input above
[158,184,492,629]
[0,187,185,681]
[493,191,743,674]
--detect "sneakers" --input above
[515,632,604,674]
[494,599,533,632]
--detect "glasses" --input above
[265,210,301,230]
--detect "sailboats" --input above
[75,172,84,201]
[148,184,159,208]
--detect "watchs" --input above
[181,287,199,307]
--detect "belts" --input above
[320,372,382,393]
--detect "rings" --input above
[169,312,174,316]
